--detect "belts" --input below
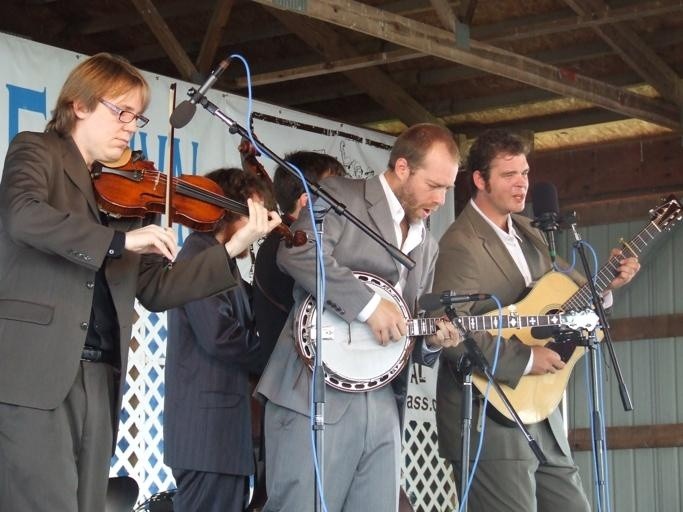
[78,345,106,363]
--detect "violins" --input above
[89,151,308,249]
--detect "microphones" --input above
[532,326,567,339]
[170,57,231,129]
[532,180,561,262]
[419,292,492,311]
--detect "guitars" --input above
[448,194,683,427]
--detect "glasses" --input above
[96,96,150,129]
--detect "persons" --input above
[162,166,277,509]
[243,147,346,512]
[249,121,466,510]
[434,121,643,510]
[0,48,283,511]
